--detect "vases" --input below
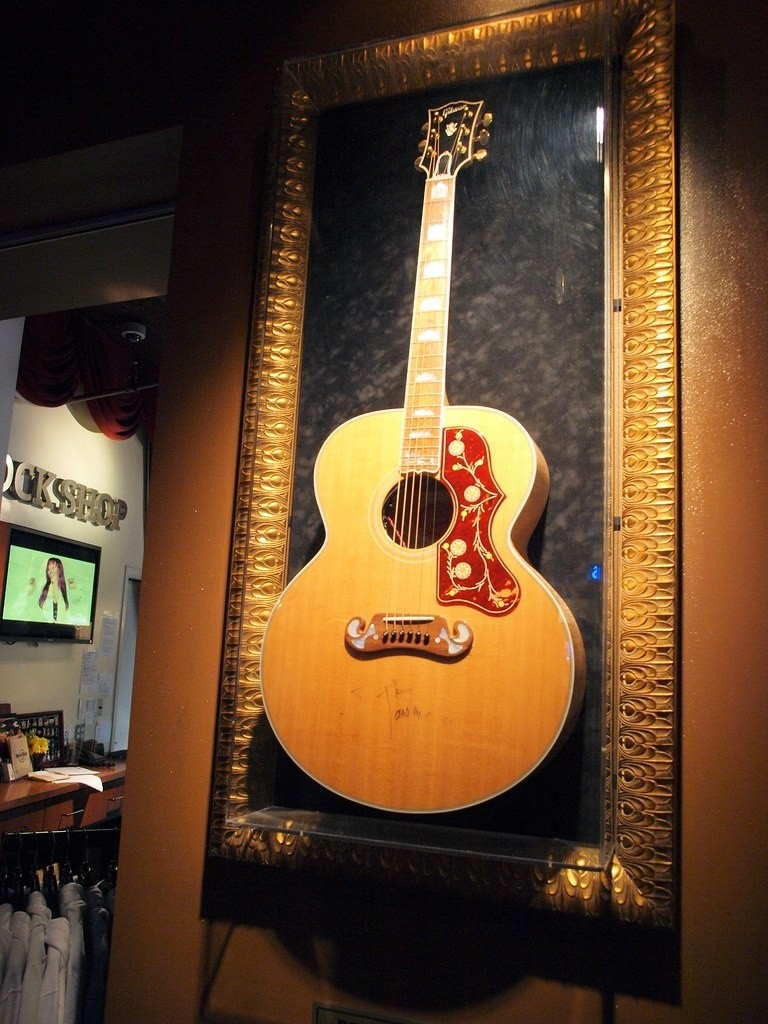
[33,752,46,770]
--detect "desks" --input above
[0,753,125,830]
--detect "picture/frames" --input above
[192,1,684,937]
[0,709,66,767]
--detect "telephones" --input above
[79,748,107,767]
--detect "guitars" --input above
[259,99,588,817]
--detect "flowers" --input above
[25,734,49,754]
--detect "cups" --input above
[65,738,82,766]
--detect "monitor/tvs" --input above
[0,521,102,644]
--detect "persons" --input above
[39,558,69,624]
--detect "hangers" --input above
[0,828,123,920]
